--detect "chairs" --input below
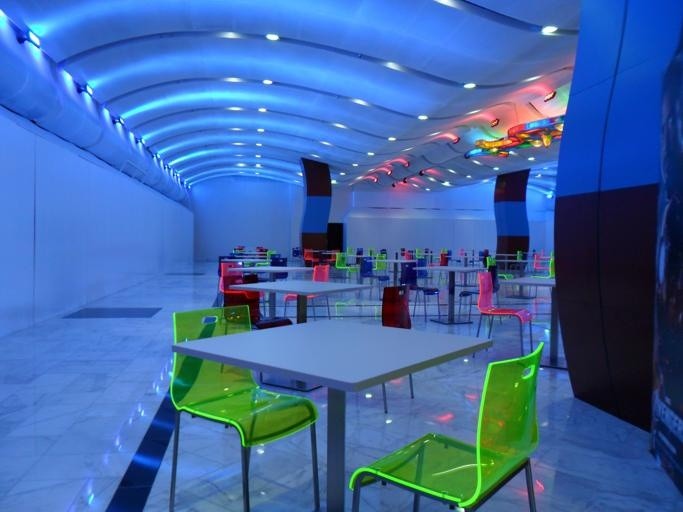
[402,263,442,324]
[370,284,414,413]
[221,278,292,329]
[219,262,264,301]
[350,341,547,511]
[458,256,500,324]
[284,265,330,322]
[170,304,322,512]
[218,244,553,299]
[476,270,534,356]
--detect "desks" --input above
[172,321,492,512]
[226,278,371,324]
